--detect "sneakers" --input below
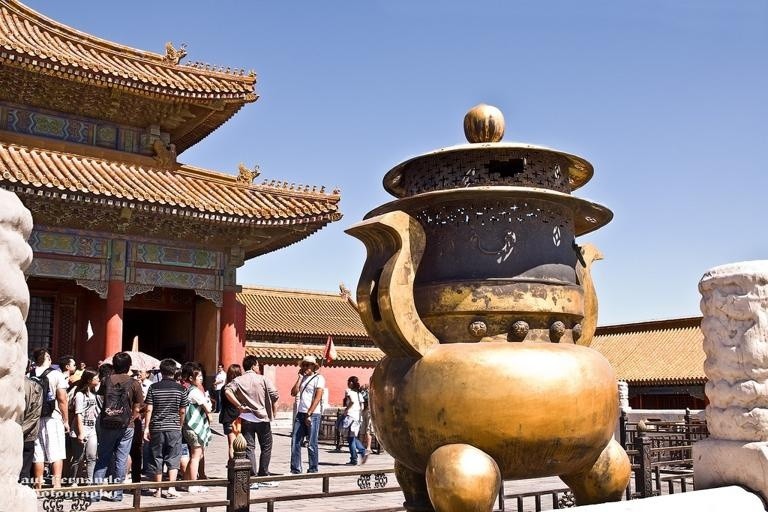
[259,481,279,488]
[249,482,259,489]
[153,491,162,498]
[361,452,368,464]
[167,492,182,498]
[345,460,356,465]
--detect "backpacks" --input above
[99,385,131,430]
[30,376,54,417]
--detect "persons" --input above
[21,346,373,501]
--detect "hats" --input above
[298,355,320,371]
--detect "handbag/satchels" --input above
[336,414,361,437]
